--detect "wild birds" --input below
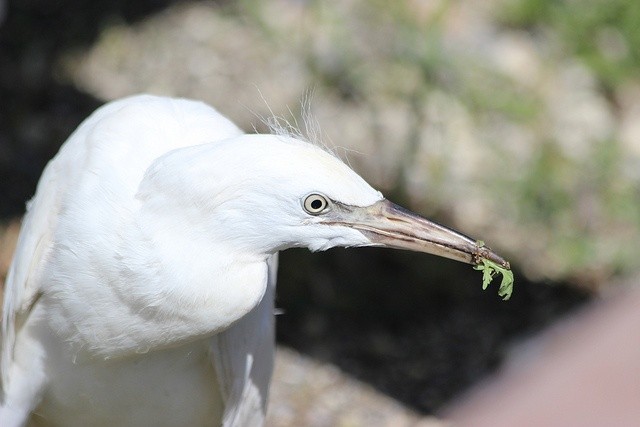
[2,87,511,427]
